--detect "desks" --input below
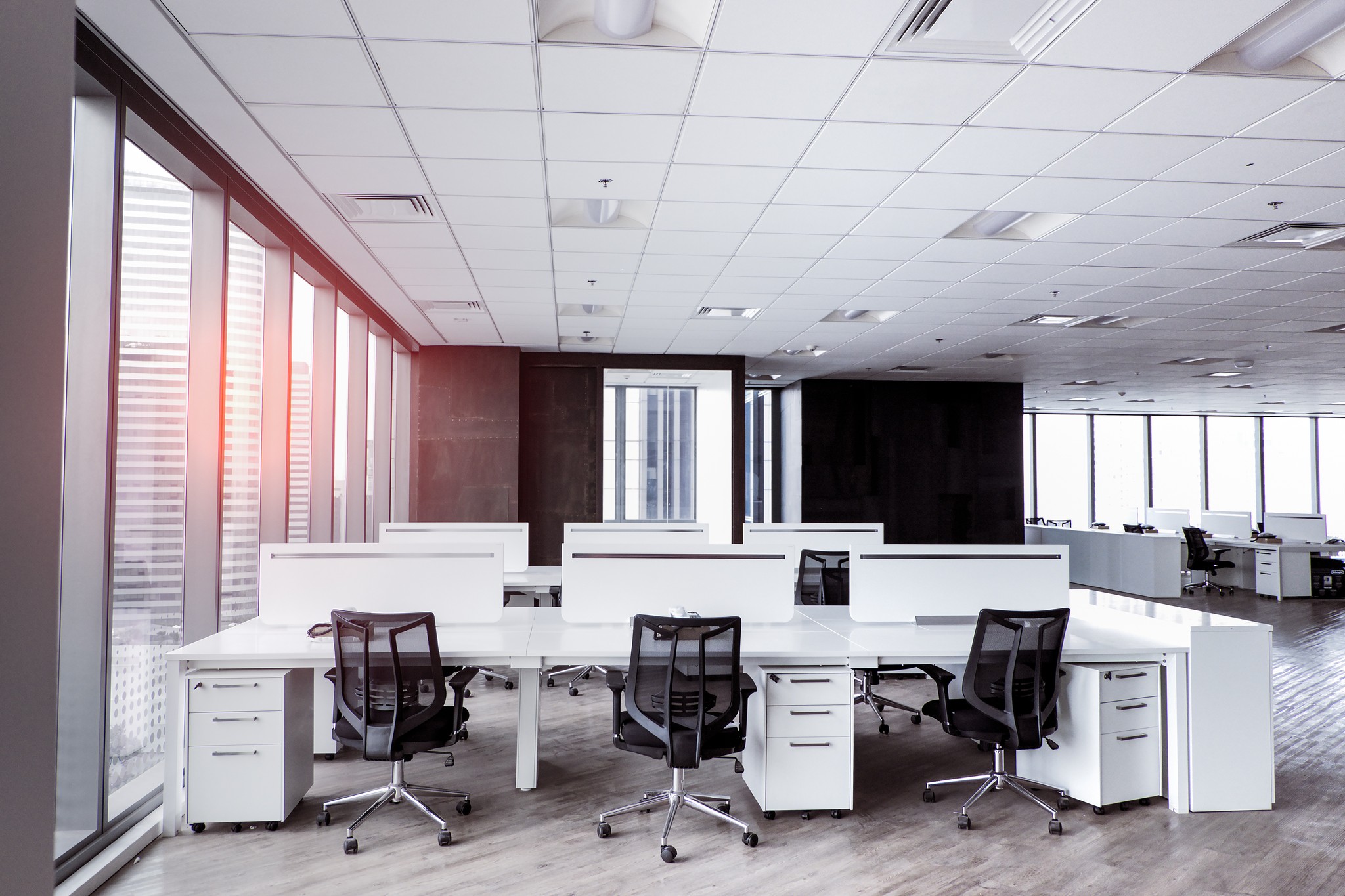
[169,533,1345,813]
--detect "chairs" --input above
[316,610,471,854]
[796,549,921,733]
[1123,524,1236,596]
[918,608,1070,836]
[597,615,758,862]
